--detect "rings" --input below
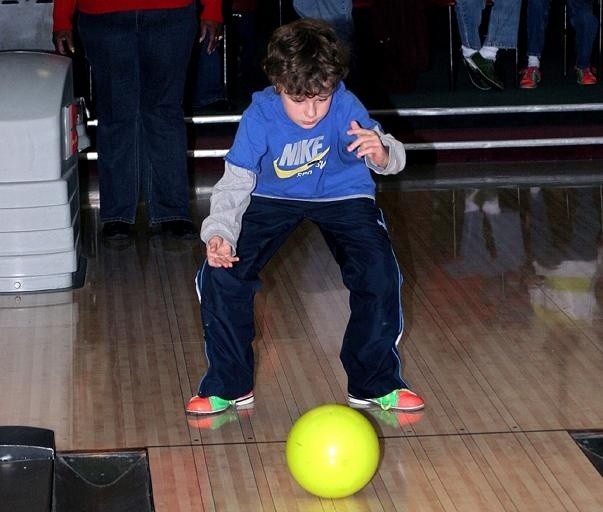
[218,35,223,41]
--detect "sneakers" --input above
[101,220,133,250]
[146,220,203,249]
[572,63,599,86]
[185,390,255,414]
[463,50,507,92]
[519,65,543,90]
[346,387,425,411]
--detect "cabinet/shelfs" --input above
[182,101,603,220]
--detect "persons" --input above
[518,1,598,89]
[456,1,521,92]
[52,0,223,252]
[182,18,428,417]
[291,1,356,93]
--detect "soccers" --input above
[285,404,380,498]
